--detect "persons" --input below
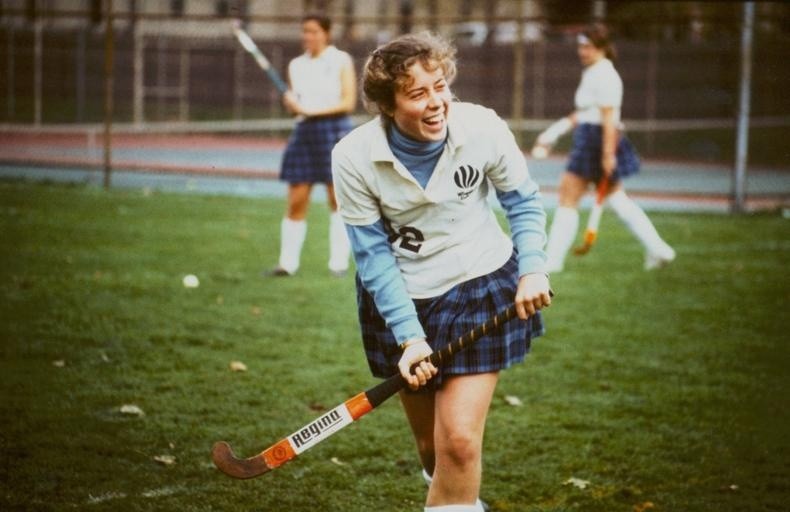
[534,19,676,273]
[330,32,551,512]
[261,13,359,279]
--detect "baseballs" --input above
[182,275,199,288]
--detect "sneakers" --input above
[643,247,675,272]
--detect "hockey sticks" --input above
[232,18,306,125]
[574,176,609,255]
[212,288,555,478]
